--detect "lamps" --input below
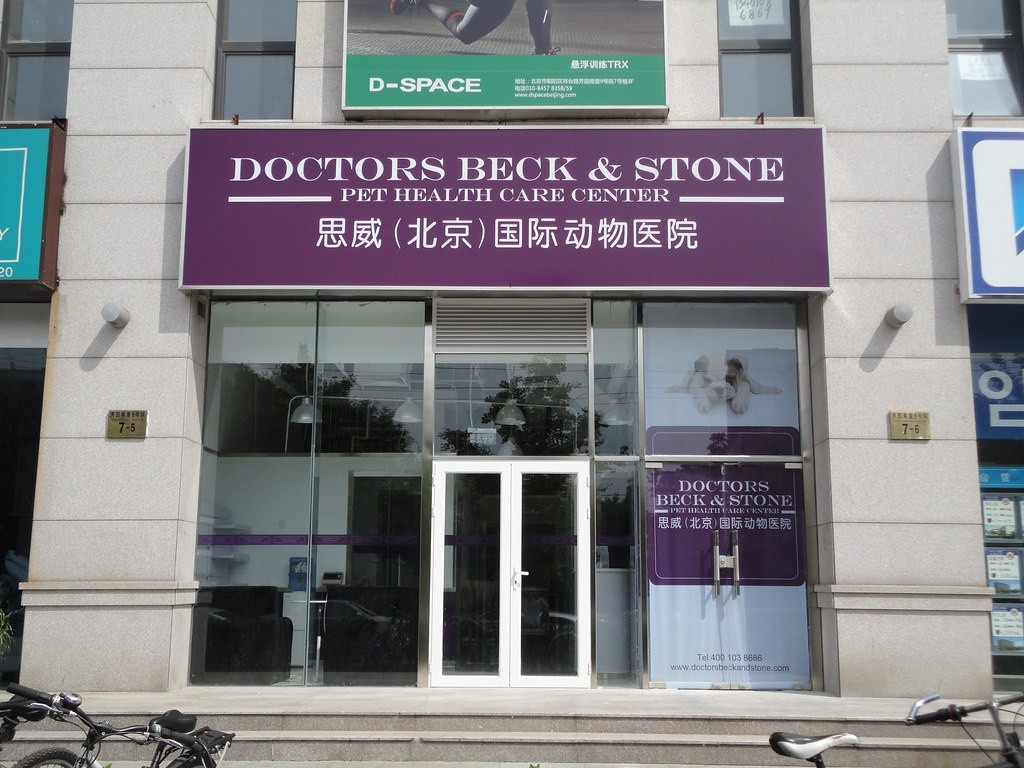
[392,364,423,424]
[100,302,129,328]
[291,363,324,424]
[495,364,526,425]
[885,303,912,328]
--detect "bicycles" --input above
[769,692,1024,768]
[0,682,236,768]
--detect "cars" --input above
[522,612,576,633]
[277,600,412,639]
